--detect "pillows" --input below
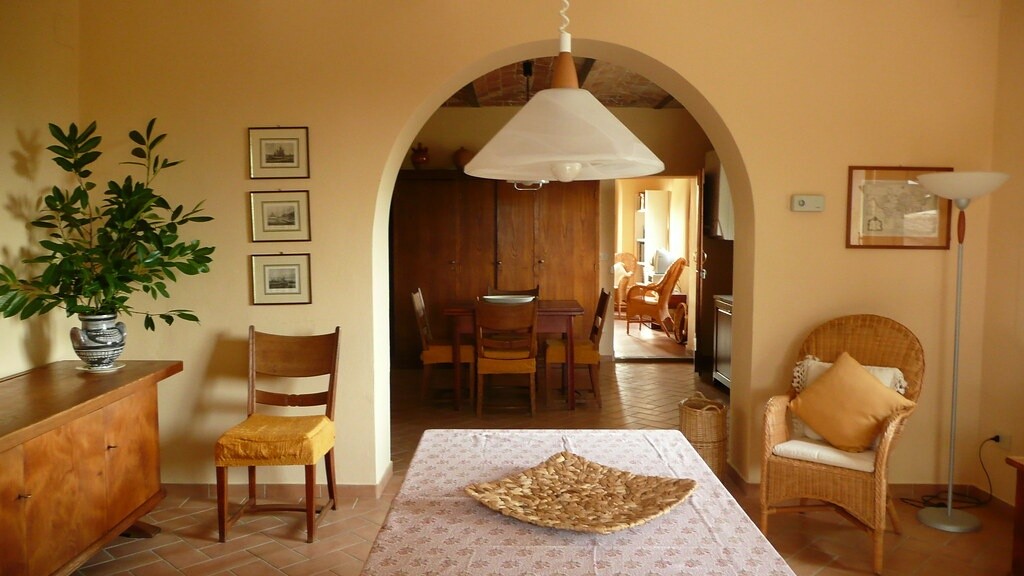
[787,351,918,452]
[790,354,909,443]
[614,261,633,290]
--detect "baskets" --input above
[679,391,727,442]
[690,441,726,483]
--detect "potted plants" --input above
[0,117,216,374]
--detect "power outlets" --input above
[992,432,1013,452]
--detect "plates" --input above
[482,295,535,304]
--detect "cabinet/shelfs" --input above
[0,360,184,576]
[392,168,601,370]
[633,189,670,285]
[711,293,732,388]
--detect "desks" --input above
[647,273,665,284]
[1004,455,1024,576]
[442,299,585,411]
[360,428,797,576]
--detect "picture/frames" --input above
[845,165,954,251]
[248,126,310,179]
[250,253,312,306]
[249,189,311,243]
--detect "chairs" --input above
[410,287,475,412]
[614,251,637,319]
[471,297,539,418]
[625,256,688,337]
[544,287,612,409]
[758,313,925,576]
[214,325,341,544]
[486,285,540,398]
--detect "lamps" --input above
[463,0,665,182]
[916,172,1011,534]
